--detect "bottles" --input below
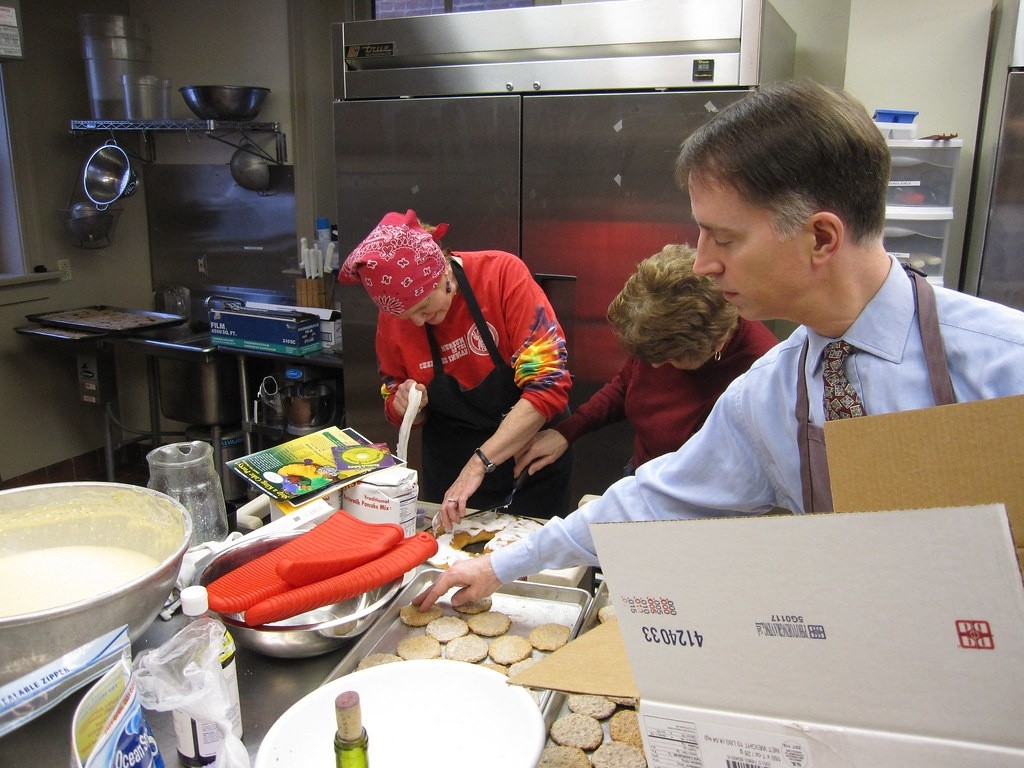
[77,13,172,122]
[173,586,242,768]
[334,726,369,768]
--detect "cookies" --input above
[535,604,650,768]
[356,587,573,709]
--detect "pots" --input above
[227,135,271,193]
[64,134,137,245]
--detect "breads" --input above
[424,512,545,582]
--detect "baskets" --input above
[56,203,124,249]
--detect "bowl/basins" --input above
[196,531,402,657]
[0,482,193,692]
[178,83,272,122]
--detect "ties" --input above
[822,340,867,421]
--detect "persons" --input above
[514,242,780,519]
[413,74,1024,613]
[337,210,576,533]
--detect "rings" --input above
[448,495,458,502]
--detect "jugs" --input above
[147,440,230,553]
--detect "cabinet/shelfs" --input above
[877,140,964,290]
[216,345,344,454]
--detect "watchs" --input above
[474,448,496,473]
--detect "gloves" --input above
[206,508,438,629]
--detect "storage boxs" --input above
[211,302,323,355]
[506,395,1024,766]
[246,300,340,347]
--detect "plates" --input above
[255,658,547,768]
